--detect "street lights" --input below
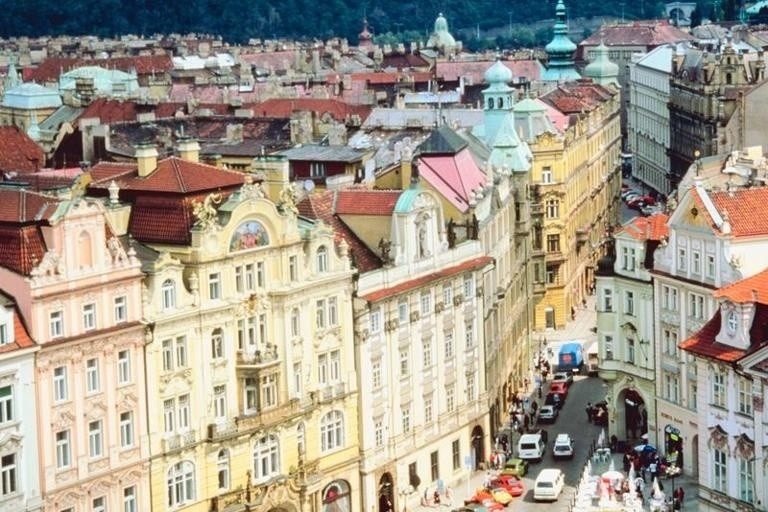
[664,464,681,512]
[397,484,414,512]
[538,333,549,399]
[639,164,645,196]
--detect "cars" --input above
[452,340,608,512]
[621,182,663,217]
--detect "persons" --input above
[585,402,594,422]
[490,352,549,469]
[597,434,686,512]
